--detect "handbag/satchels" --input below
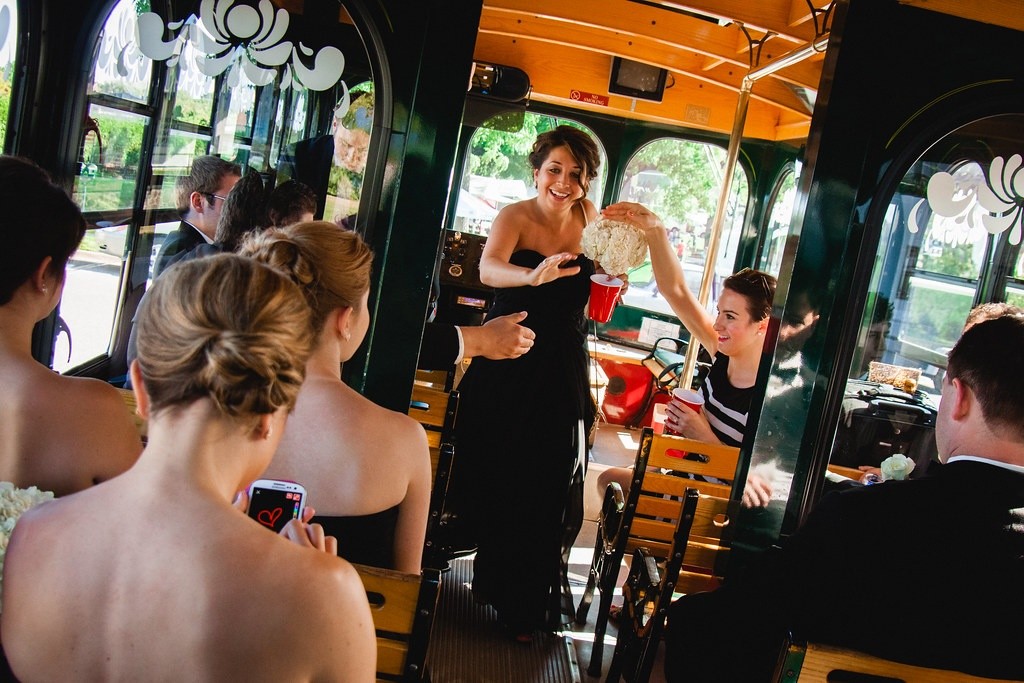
[587,390,608,450]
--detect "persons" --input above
[177,175,320,265]
[326,211,537,368]
[0,152,143,508]
[424,122,630,642]
[230,222,433,578]
[857,293,892,380]
[758,307,1024,682]
[597,199,781,627]
[1,253,378,683]
[272,89,383,227]
[143,156,247,296]
[736,266,825,505]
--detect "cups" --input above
[665,388,705,436]
[588,274,624,323]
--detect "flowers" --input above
[0,481,60,616]
[580,213,649,277]
[881,453,916,480]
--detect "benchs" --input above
[114,388,458,577]
[767,630,1024,683]
[624,336,713,430]
[414,362,456,393]
[605,483,730,683]
[343,551,445,683]
[411,384,461,447]
[575,425,740,679]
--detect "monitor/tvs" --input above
[607,55,668,104]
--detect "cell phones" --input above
[246,476,307,537]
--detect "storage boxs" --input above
[651,402,671,434]
